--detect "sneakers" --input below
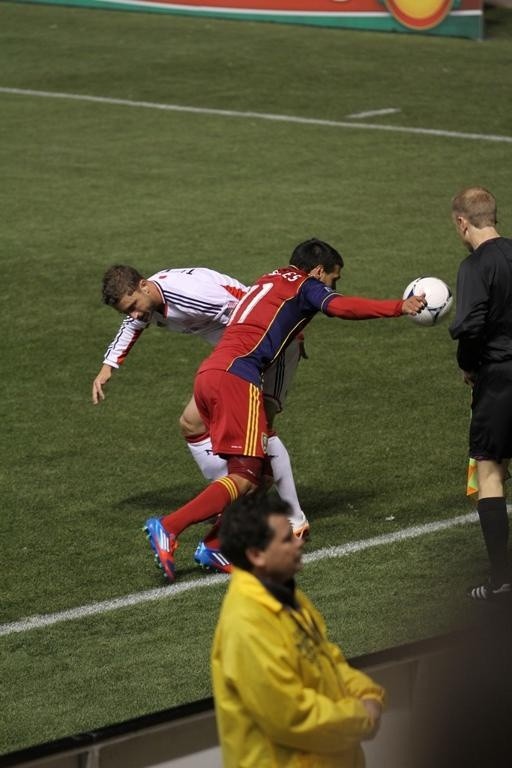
[141,515,177,583]
[194,539,233,574]
[289,511,312,537]
[467,581,512,602]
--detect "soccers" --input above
[403,275,455,329]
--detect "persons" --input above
[141,236,430,584]
[91,262,312,543]
[210,490,391,768]
[445,184,511,607]
